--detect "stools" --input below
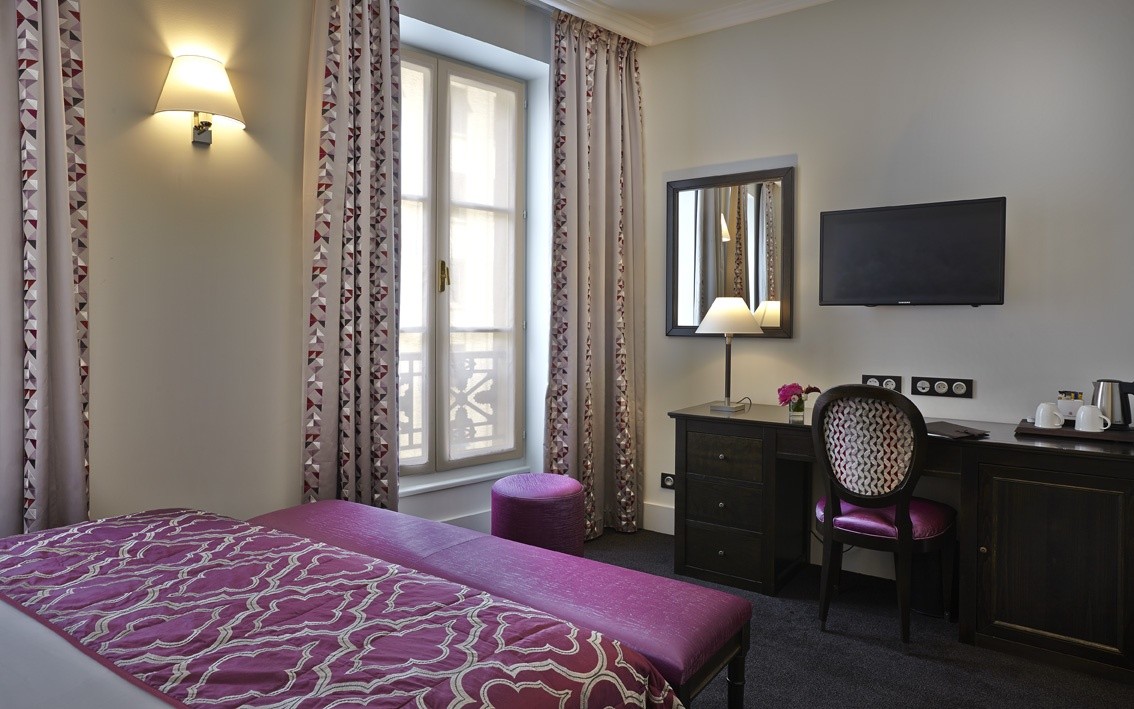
[491,475,584,558]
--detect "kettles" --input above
[1090,379,1134,431]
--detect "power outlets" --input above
[909,377,974,400]
[862,374,902,396]
[660,473,676,491]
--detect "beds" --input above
[2,508,688,709]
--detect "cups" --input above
[1058,399,1084,421]
[1075,405,1111,433]
[1034,402,1064,429]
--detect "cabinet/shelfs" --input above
[665,402,1134,685]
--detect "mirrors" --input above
[664,167,793,339]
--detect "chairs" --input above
[812,384,961,645]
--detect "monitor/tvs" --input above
[817,194,1008,311]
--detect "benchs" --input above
[247,498,752,709]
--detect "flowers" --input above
[777,383,821,408]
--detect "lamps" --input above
[151,52,246,148]
[720,211,731,244]
[752,300,783,327]
[696,296,763,414]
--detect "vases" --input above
[788,397,804,417]
